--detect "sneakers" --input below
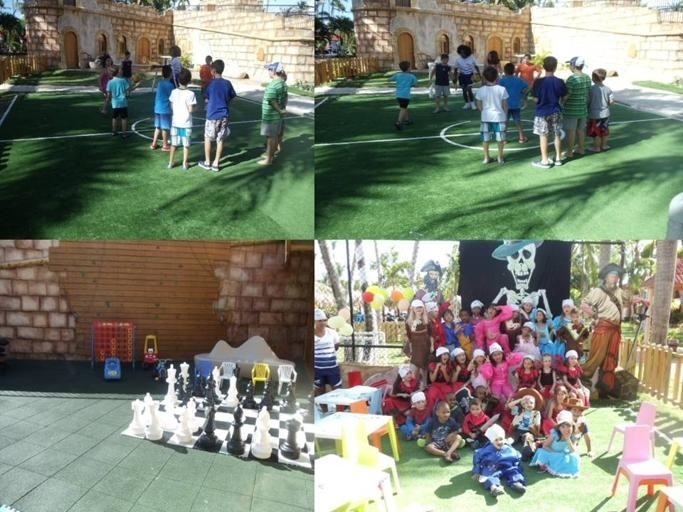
[532,158,562,168]
[519,136,528,143]
[462,102,477,110]
[198,161,220,170]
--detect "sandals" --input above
[395,120,413,128]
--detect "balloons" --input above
[363,284,414,311]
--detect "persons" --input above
[198,59,237,171]
[149,65,176,152]
[276,69,287,80]
[410,260,444,309]
[387,61,420,129]
[168,70,198,170]
[168,45,183,83]
[200,55,213,93]
[97,54,116,114]
[314,309,343,409]
[585,263,635,402]
[102,65,133,138]
[425,44,614,169]
[256,62,289,166]
[383,279,591,499]
[122,51,133,97]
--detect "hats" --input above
[314,309,327,320]
[265,62,283,73]
[598,263,626,280]
[566,56,583,69]
[398,299,579,444]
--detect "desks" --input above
[313,384,403,511]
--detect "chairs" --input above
[276,365,297,394]
[605,399,683,512]
[251,362,271,388]
[215,360,237,382]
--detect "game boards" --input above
[120,388,313,471]
